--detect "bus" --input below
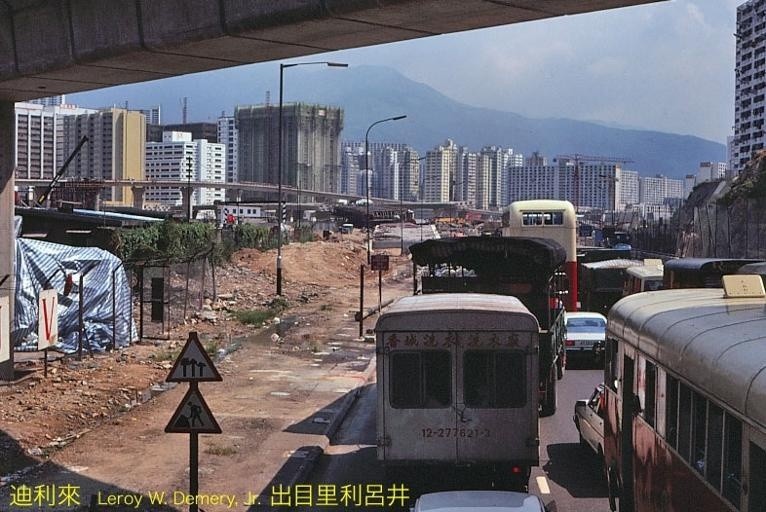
[501,199,577,311]
[602,273,764,511]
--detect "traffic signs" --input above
[370,255,388,271]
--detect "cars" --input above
[563,311,607,357]
[572,384,606,480]
[409,489,547,512]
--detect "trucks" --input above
[372,291,540,491]
[409,235,567,415]
[576,243,765,316]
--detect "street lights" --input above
[400,156,426,256]
[275,61,349,296]
[365,115,407,263]
